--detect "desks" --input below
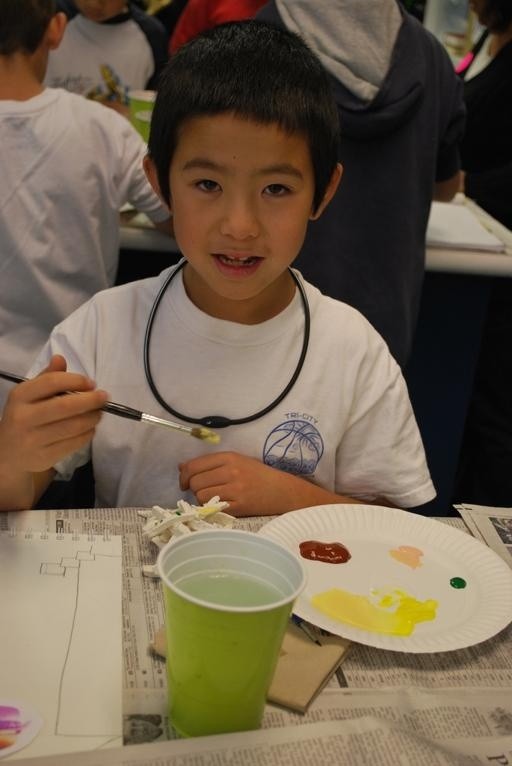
[1,507,512,766]
[121,192,510,517]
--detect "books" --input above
[150,610,351,716]
[0,526,127,764]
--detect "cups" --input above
[125,89,160,145]
[158,531,307,738]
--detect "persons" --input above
[0,0,512,519]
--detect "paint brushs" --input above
[0,370,221,446]
[292,614,321,647]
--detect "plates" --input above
[258,504,510,653]
